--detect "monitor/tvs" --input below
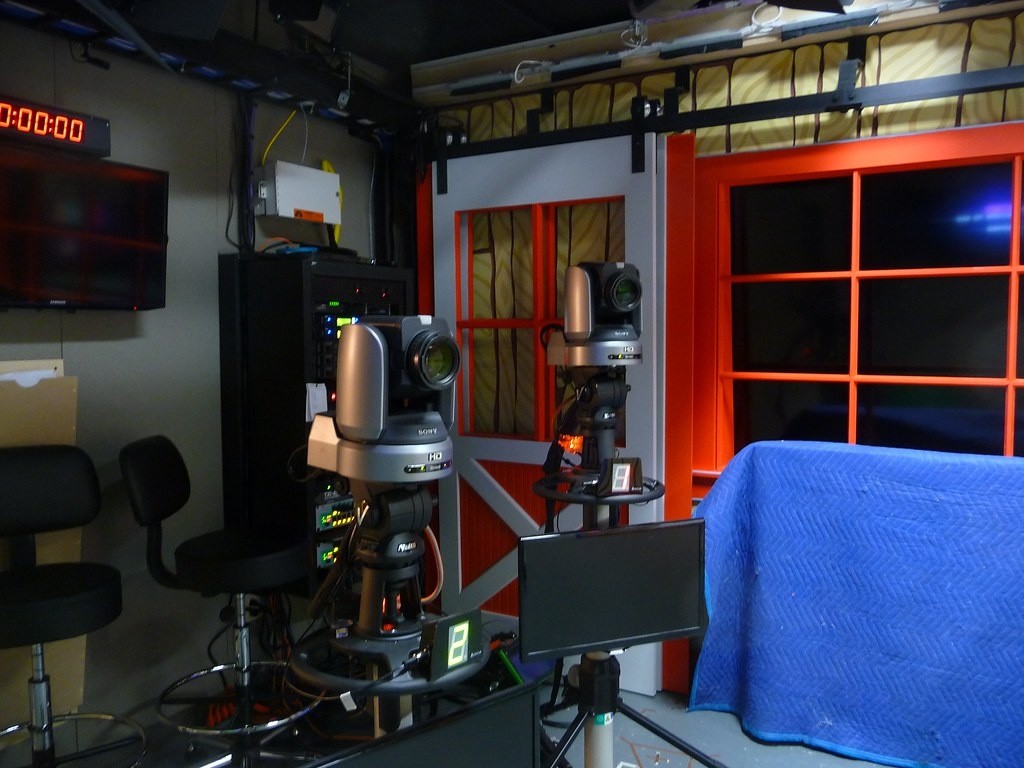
[0,139,169,311]
[518,518,705,664]
[298,680,542,768]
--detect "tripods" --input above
[541,652,731,768]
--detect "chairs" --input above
[0,444,146,767]
[120,435,325,768]
